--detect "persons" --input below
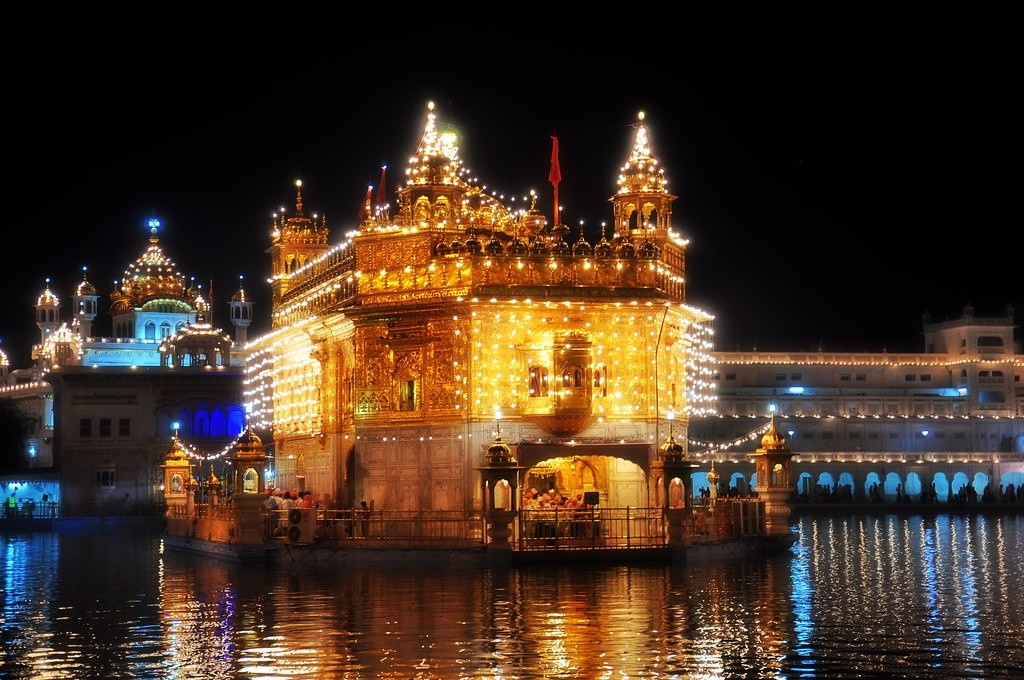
[4,494,16,519]
[698,482,1024,506]
[194,480,371,538]
[523,486,600,541]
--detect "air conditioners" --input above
[287,507,316,544]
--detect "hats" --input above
[549,489,555,493]
[538,497,544,502]
